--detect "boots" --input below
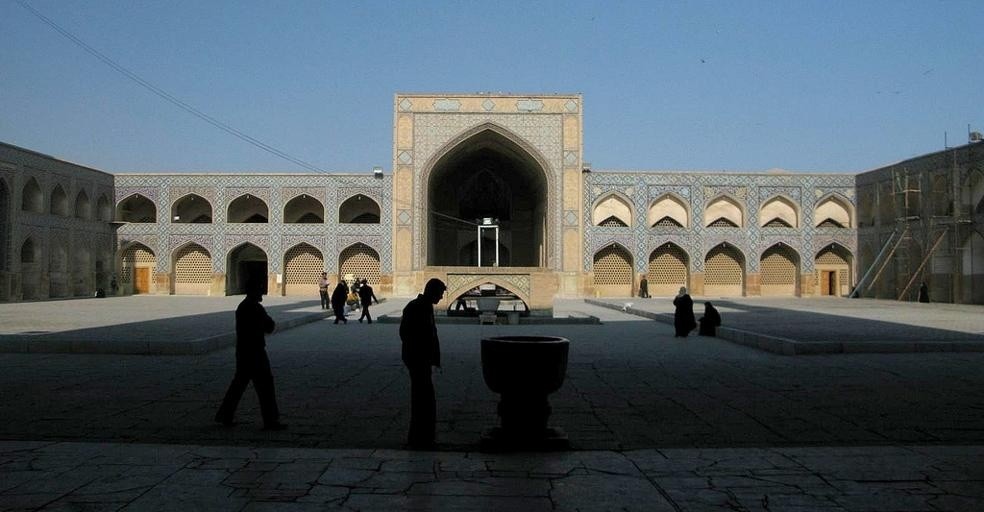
[333,318,347,324]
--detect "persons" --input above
[399,279,455,452]
[215,274,289,432]
[673,286,721,337]
[319,272,378,324]
[640,275,649,298]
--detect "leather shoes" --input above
[214,417,281,431]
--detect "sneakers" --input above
[359,318,372,324]
[407,432,435,445]
